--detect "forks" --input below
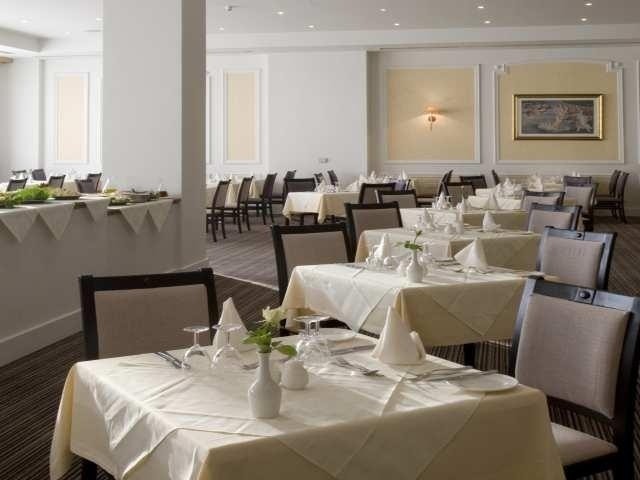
[399,364,499,385]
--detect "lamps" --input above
[422,106,447,132]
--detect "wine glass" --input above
[180,324,211,378]
[292,311,333,365]
[212,322,244,375]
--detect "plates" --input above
[445,371,520,394]
[312,328,357,342]
[50,194,84,199]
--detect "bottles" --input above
[159,183,168,197]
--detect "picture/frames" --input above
[510,92,605,142]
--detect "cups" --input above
[307,165,581,283]
[279,358,309,390]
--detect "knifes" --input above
[155,350,190,369]
